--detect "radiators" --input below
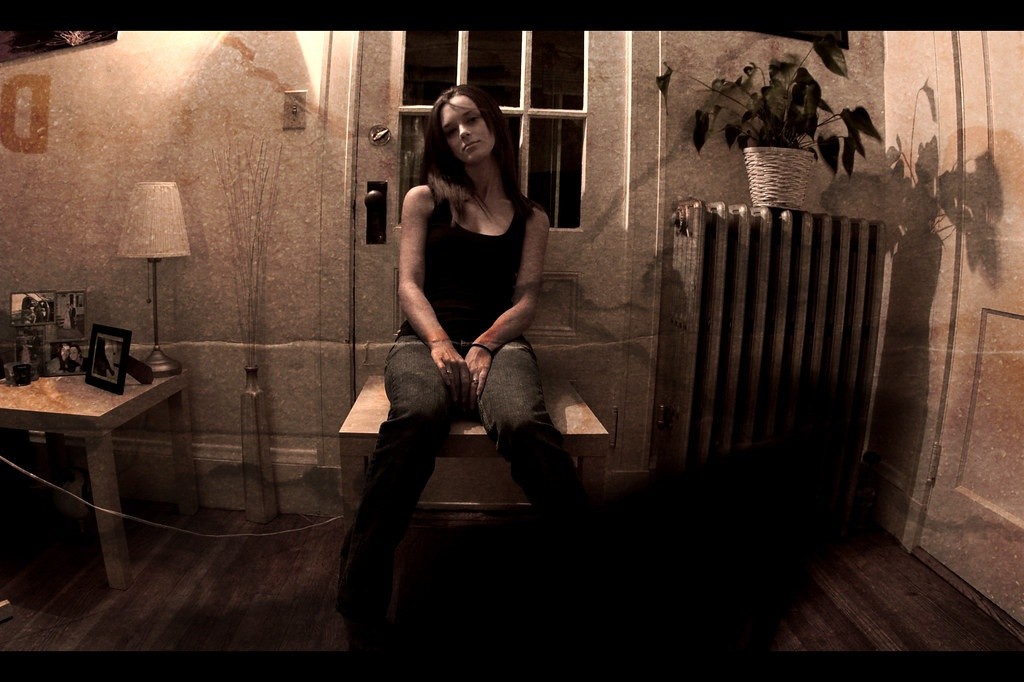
[655,197,895,535]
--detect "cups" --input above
[4,361,39,387]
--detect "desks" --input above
[0,368,197,591]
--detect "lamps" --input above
[116,181,191,378]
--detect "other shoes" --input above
[341,616,388,650]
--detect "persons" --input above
[21,328,39,363]
[94,337,115,377]
[61,302,77,329]
[59,342,88,372]
[337,84,594,650]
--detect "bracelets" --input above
[470,344,494,359]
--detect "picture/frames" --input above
[42,339,90,378]
[85,323,132,395]
[10,289,57,327]
[56,288,87,339]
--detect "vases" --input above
[239,365,280,533]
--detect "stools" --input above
[337,376,611,559]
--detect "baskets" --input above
[742,145,819,212]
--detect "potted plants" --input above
[652,40,883,210]
